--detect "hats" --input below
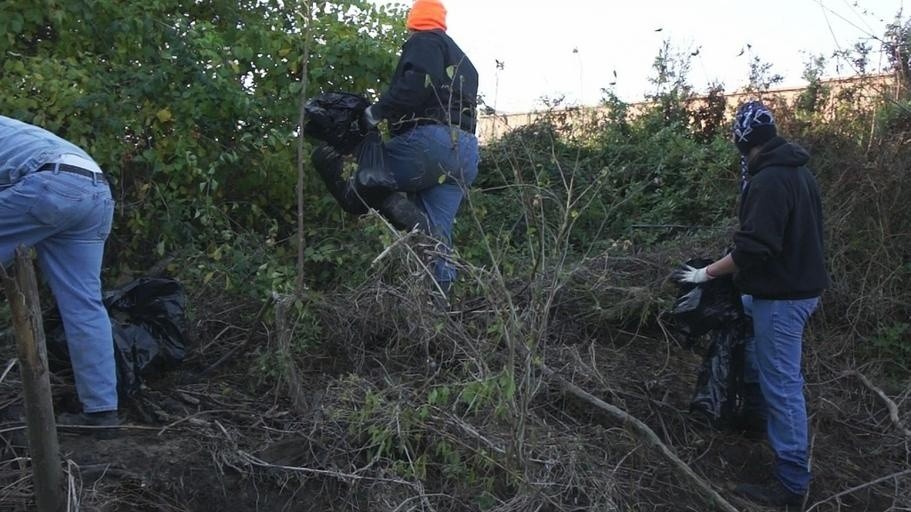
[733,101,777,156]
[406,0,447,31]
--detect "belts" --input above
[38,163,105,180]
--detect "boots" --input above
[434,281,454,311]
[382,192,427,234]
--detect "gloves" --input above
[680,264,716,284]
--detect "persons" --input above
[671,100,830,505]
[0,113,122,440]
[363,0,482,311]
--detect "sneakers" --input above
[723,410,767,439]
[59,411,118,438]
[734,481,806,511]
